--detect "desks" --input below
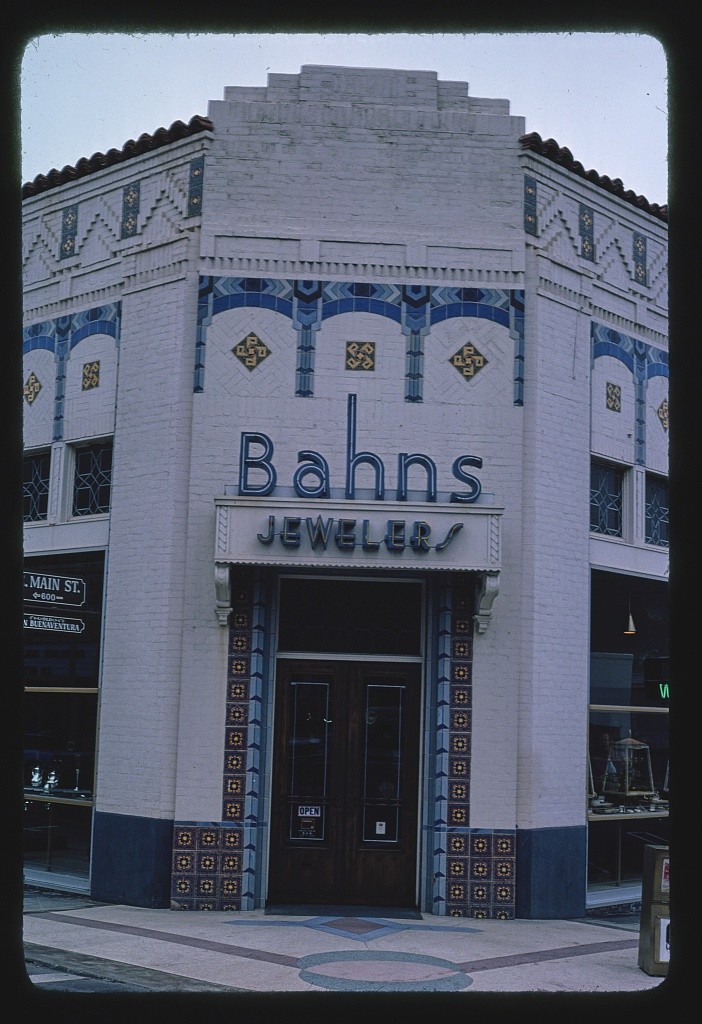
[23,792,92,872]
[587,802,669,887]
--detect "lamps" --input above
[621,590,637,635]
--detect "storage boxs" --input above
[637,897,670,976]
[642,844,670,903]
